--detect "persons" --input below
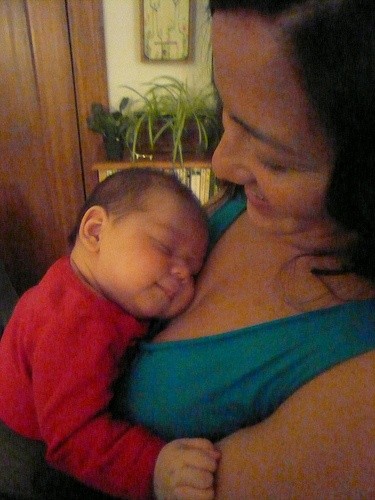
[0,167,223,500]
[42,0,375,500]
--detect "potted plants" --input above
[86,75,222,166]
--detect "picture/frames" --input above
[140,0,193,63]
